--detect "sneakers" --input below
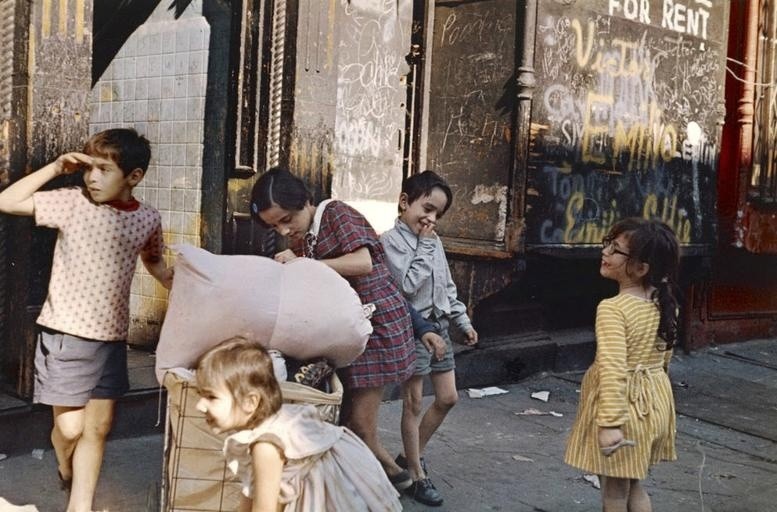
[388,455,443,506]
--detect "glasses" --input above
[603,240,630,258]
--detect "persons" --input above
[378,169,480,508]
[0,127,177,511]
[560,218,679,511]
[194,334,404,512]
[248,167,416,492]
